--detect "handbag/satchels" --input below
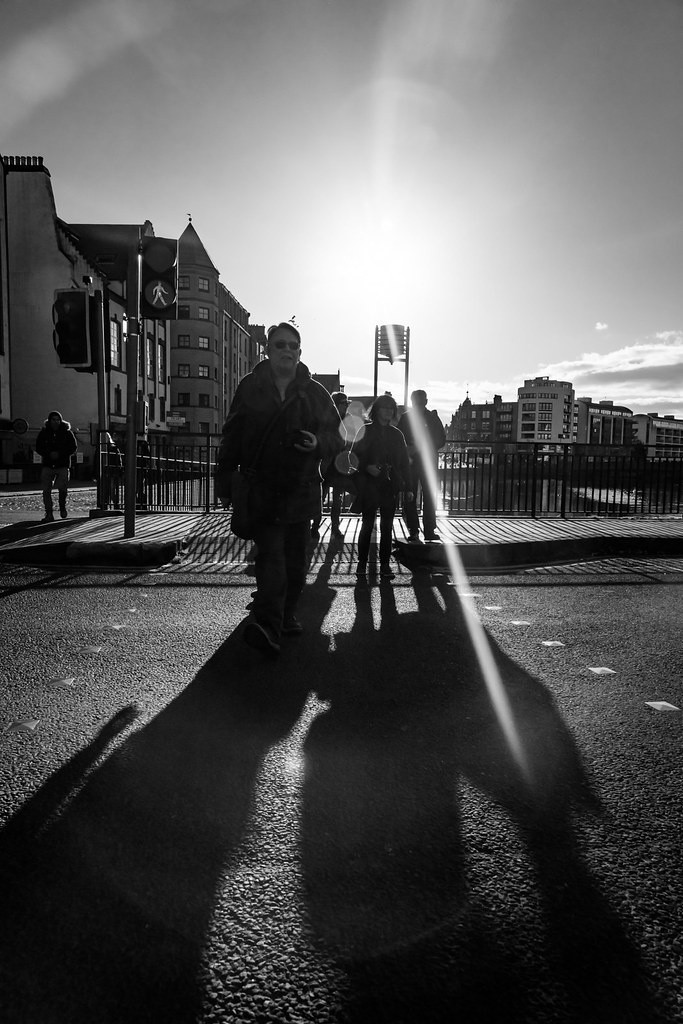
[230,467,276,541]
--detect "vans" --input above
[464,447,494,466]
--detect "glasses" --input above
[276,341,298,350]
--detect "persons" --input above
[217,326,345,669]
[312,391,349,539]
[397,391,447,540]
[92,432,123,510]
[36,412,79,524]
[136,436,151,510]
[348,395,410,578]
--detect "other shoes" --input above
[41,515,54,523]
[358,550,369,560]
[425,533,440,540]
[332,529,341,536]
[407,532,419,541]
[244,622,280,651]
[381,566,395,578]
[60,506,67,518]
[283,616,303,632]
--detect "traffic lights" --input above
[51,288,92,368]
[140,236,179,323]
